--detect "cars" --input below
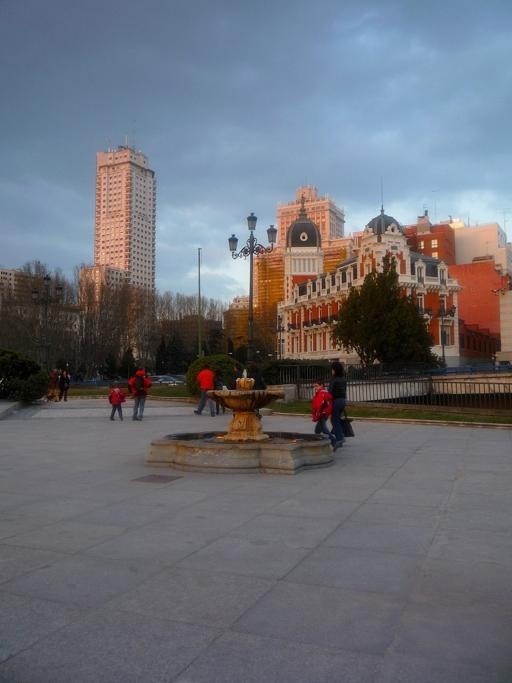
[148,375,184,387]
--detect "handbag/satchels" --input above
[64,384,71,388]
[341,418,354,437]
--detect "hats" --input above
[114,381,120,385]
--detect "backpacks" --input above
[134,375,147,391]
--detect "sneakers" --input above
[194,409,225,417]
[331,438,345,452]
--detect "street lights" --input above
[227,210,277,368]
[429,293,456,364]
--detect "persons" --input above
[105,380,126,421]
[47,367,60,402]
[192,363,218,416]
[127,365,153,421]
[309,379,340,452]
[213,369,227,415]
[327,360,349,447]
[54,370,71,401]
